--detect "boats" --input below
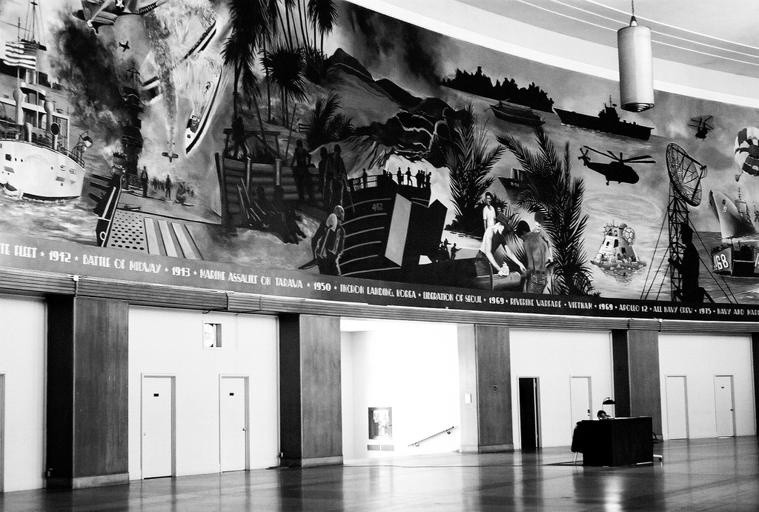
[553,95,654,141]
[706,174,758,282]
[488,99,545,127]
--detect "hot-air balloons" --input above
[733,126,759,181]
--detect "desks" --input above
[571,416,654,467]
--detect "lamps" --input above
[618,1,654,113]
[601,397,616,410]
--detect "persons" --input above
[597,410,611,420]
[140,165,190,206]
[291,139,347,276]
[360,166,431,189]
[475,192,551,295]
[668,221,699,303]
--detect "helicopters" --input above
[689,113,714,140]
[577,143,657,187]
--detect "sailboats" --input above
[0,0,90,201]
